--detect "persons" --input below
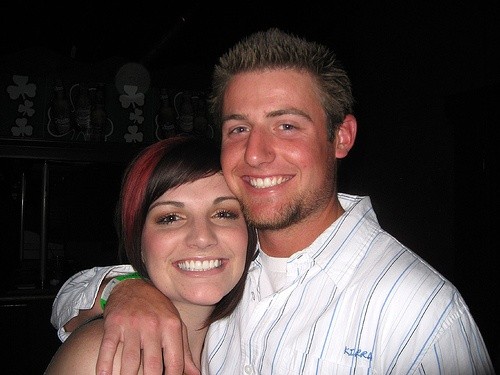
[49,30,494,375]
[45,138,257,375]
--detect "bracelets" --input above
[100,274,142,312]
